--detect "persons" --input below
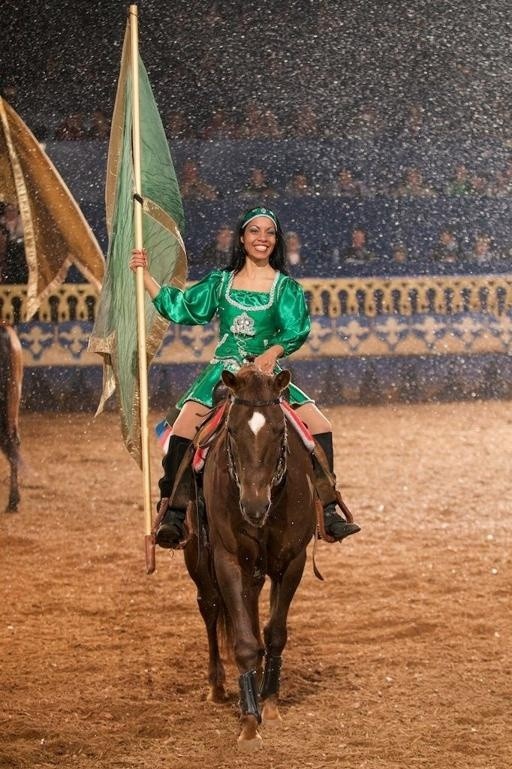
[130,205,361,546]
[2,204,108,277]
[166,94,511,275]
[14,97,111,140]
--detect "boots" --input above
[156,434,193,547]
[312,432,360,540]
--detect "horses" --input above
[183,365,317,756]
[0,319,25,514]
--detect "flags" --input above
[1,88,106,318]
[89,12,189,466]
